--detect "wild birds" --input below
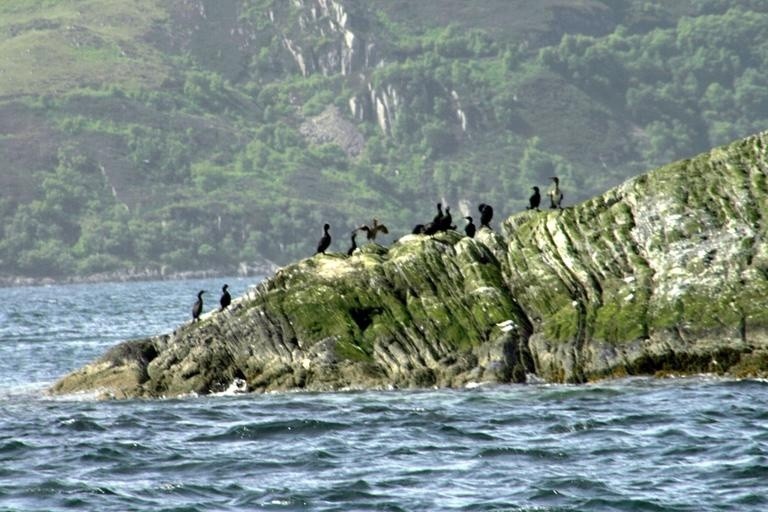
[411,201,457,236]
[353,215,389,244]
[218,283,232,313]
[461,216,476,239]
[478,202,493,231]
[546,176,564,210]
[191,289,206,324]
[525,186,543,212]
[313,223,332,257]
[346,233,357,258]
